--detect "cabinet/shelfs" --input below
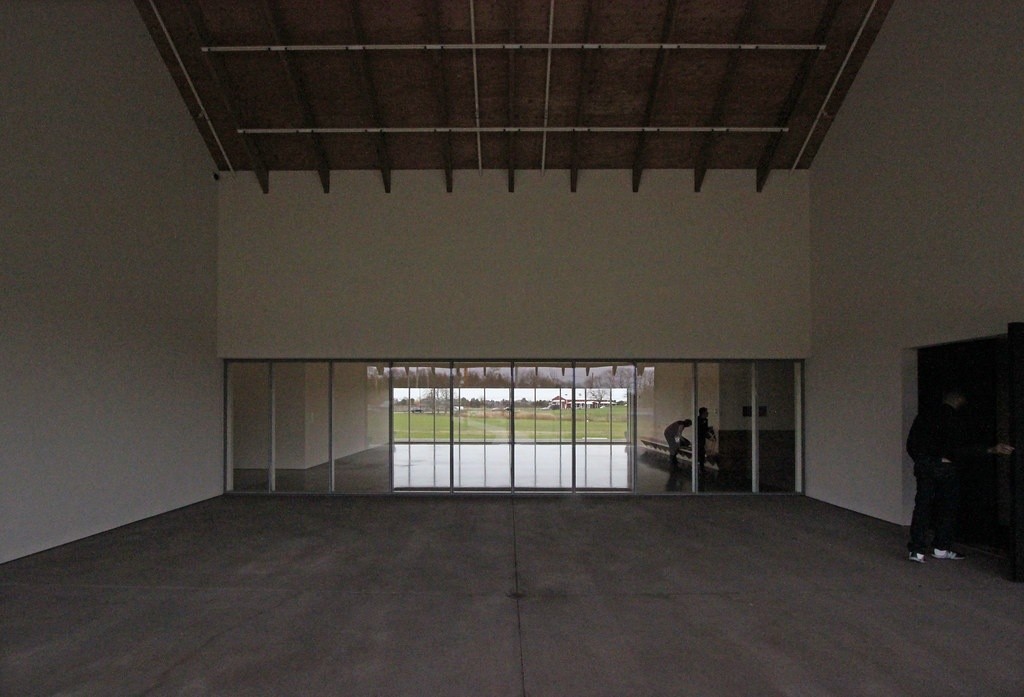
[908,321,1024,581]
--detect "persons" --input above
[698,408,714,475]
[906,388,1016,563]
[664,419,692,464]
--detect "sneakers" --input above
[930,542,966,560]
[908,550,926,563]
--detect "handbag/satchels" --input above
[705,432,715,455]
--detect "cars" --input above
[409,407,425,413]
[543,405,561,410]
